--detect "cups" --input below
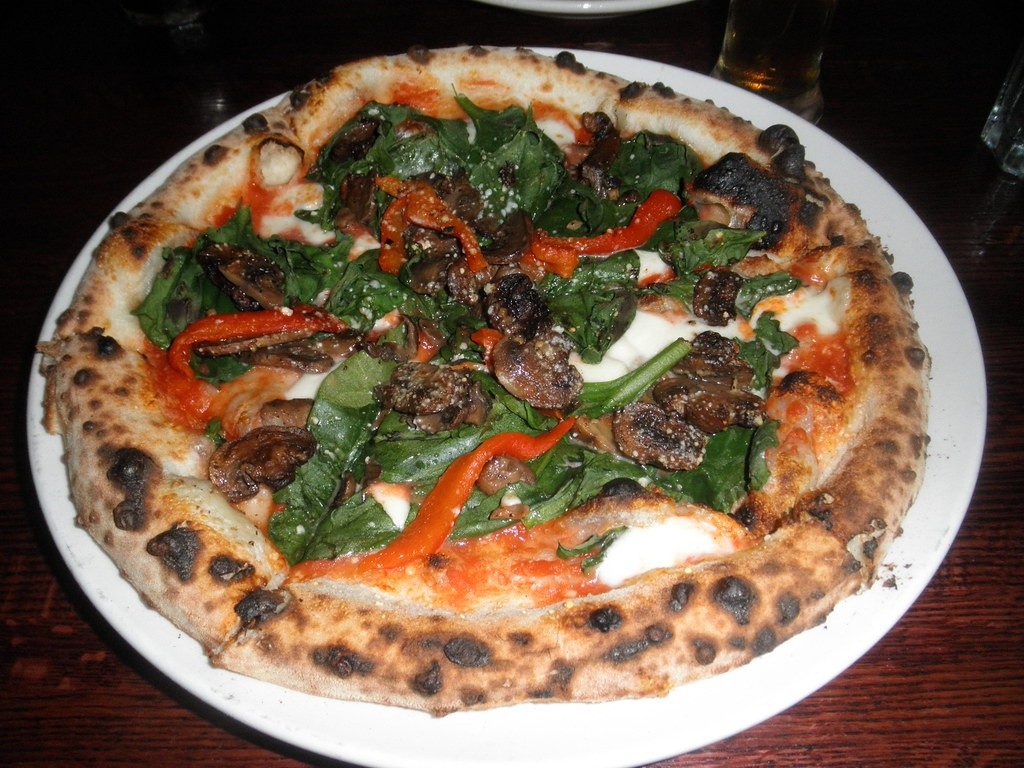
[709,0,828,129]
[981,42,1024,175]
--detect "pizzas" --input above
[37,42,933,717]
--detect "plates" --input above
[25,45,990,768]
[475,0,696,21]
